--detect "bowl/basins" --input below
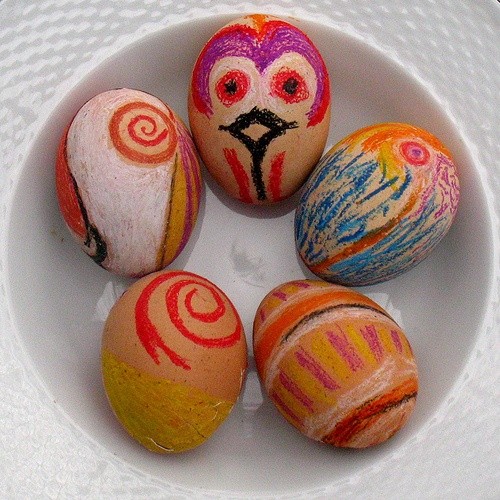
[0,6,497,498]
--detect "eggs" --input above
[253,279,420,449]
[101,270,250,455]
[293,122,460,288]
[55,87,204,279]
[186,13,334,208]
